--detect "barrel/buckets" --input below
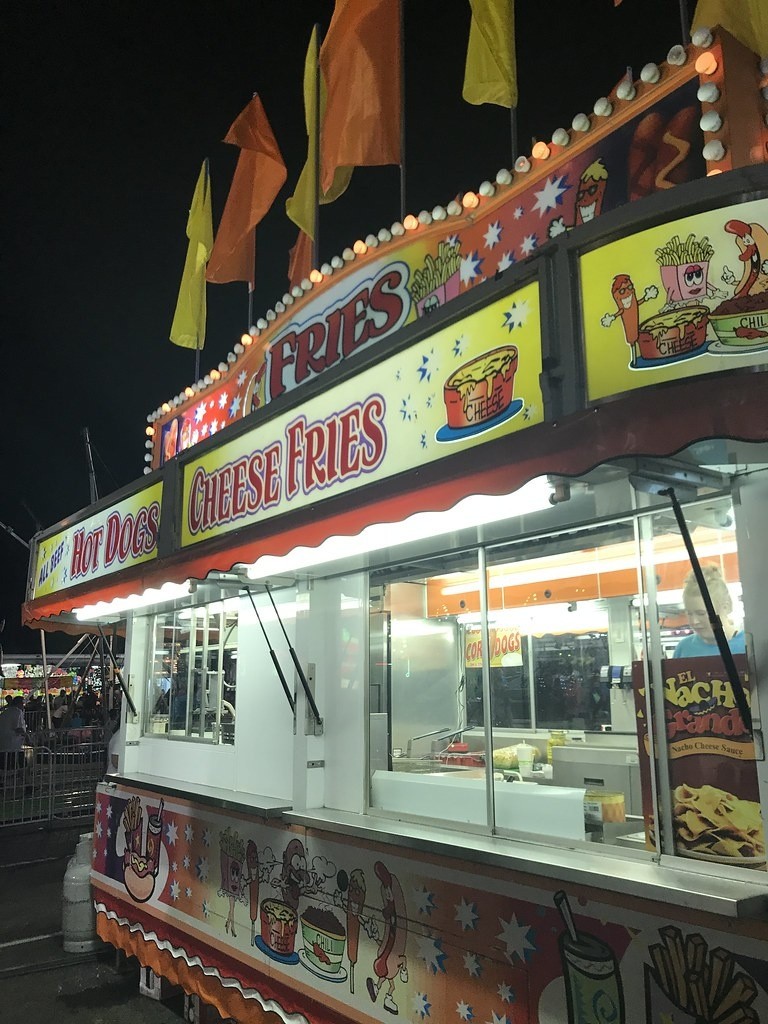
[583,790,625,832]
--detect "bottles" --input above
[149,714,168,733]
[547,729,585,764]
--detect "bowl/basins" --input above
[541,767,552,779]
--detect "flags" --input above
[289,227,316,293]
[166,156,213,383]
[287,25,355,282]
[320,0,404,224]
[455,0,516,165]
[203,90,288,328]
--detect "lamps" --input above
[479,181,495,197]
[531,141,550,160]
[572,113,590,132]
[706,168,722,177]
[616,80,636,100]
[551,128,570,146]
[666,45,687,66]
[142,204,434,476]
[463,191,480,209]
[695,52,718,75]
[699,109,723,132]
[446,200,463,216]
[701,139,725,161]
[431,205,447,220]
[641,63,661,83]
[515,155,531,173]
[594,97,613,117]
[692,27,713,48]
[496,168,513,185]
[697,81,721,103]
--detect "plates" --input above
[648,830,766,865]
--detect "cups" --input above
[517,747,534,777]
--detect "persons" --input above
[673,559,746,658]
[0,690,124,774]
